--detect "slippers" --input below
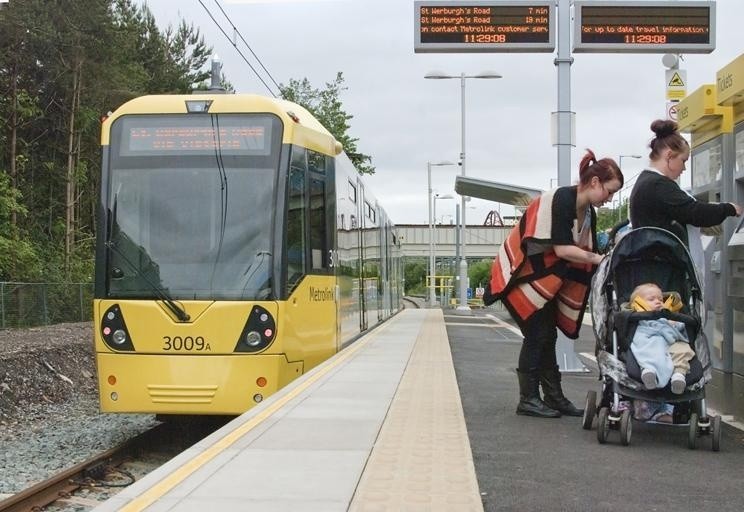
[645,412,690,426]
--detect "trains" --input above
[93,53,403,423]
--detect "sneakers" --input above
[670,373,687,394]
[641,368,657,390]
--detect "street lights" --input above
[619,155,641,222]
[423,70,502,310]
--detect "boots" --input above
[515,365,585,418]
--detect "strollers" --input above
[583,219,721,449]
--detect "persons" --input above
[483,148,624,418]
[630,119,742,247]
[630,282,695,395]
